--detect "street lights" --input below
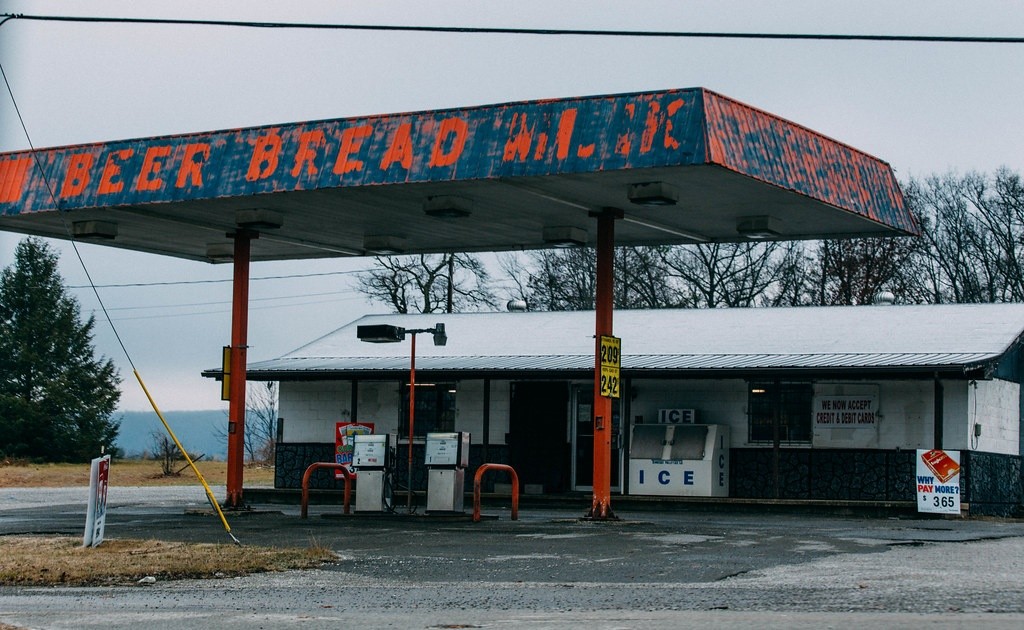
[357,323,448,514]
